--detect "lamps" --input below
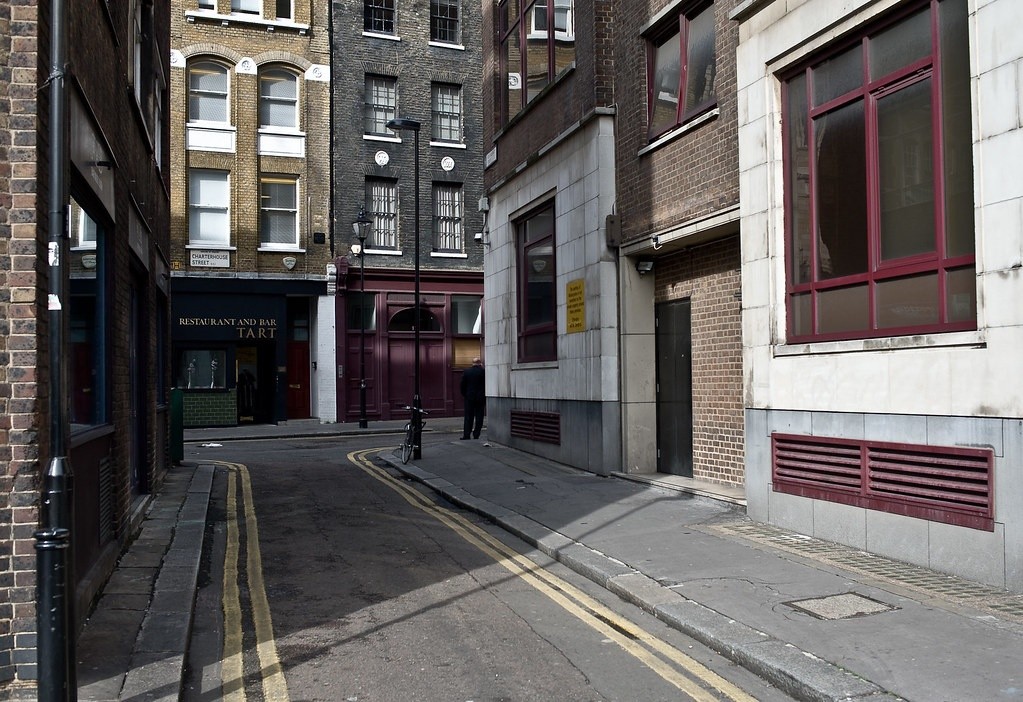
[635,260,654,278]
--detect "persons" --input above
[460,358,485,440]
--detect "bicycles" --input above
[398,405,430,465]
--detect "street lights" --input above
[385,119,426,461]
[349,203,376,432]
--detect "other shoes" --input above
[460,437,470,440]
